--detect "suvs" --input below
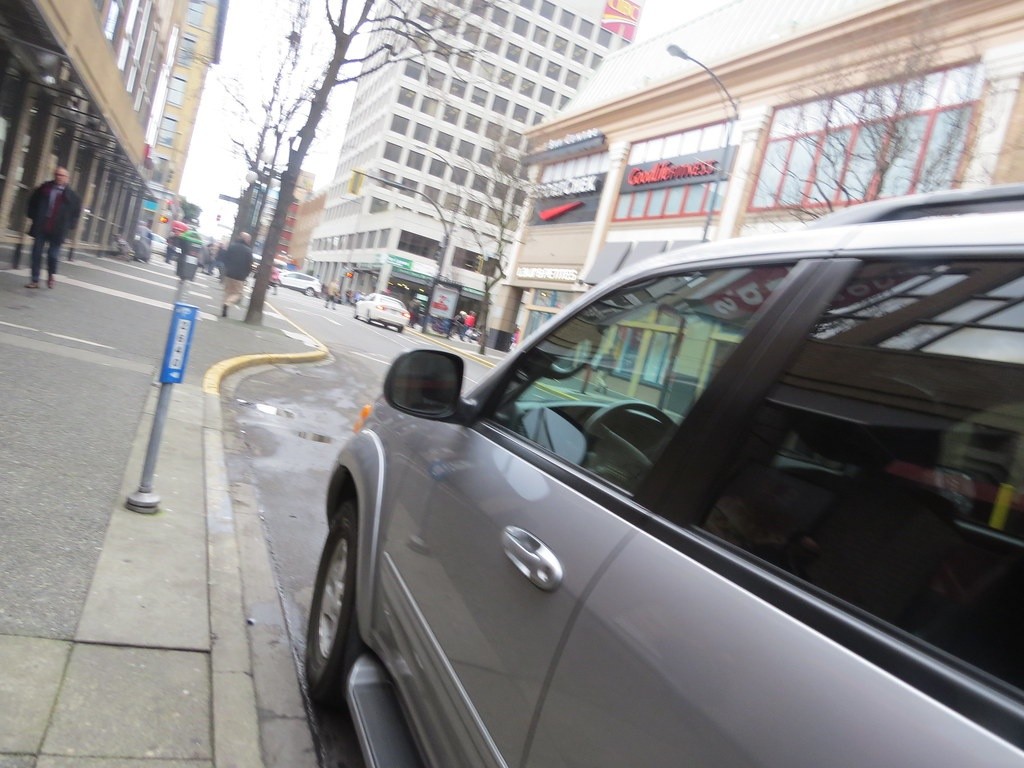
[303,184,1024,768]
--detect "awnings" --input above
[764,385,1011,437]
[584,240,708,285]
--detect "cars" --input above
[352,293,410,333]
[151,232,182,260]
[250,253,322,298]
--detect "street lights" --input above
[352,142,460,334]
[658,44,741,414]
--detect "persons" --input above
[165,235,176,264]
[206,242,226,277]
[509,328,519,350]
[407,298,420,328]
[269,264,280,295]
[137,220,151,238]
[197,243,214,272]
[463,311,476,342]
[23,165,82,288]
[595,348,615,394]
[345,289,365,303]
[222,232,253,317]
[450,310,467,342]
[322,279,340,311]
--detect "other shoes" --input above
[24,283,40,289]
[47,275,55,289]
[222,304,228,317]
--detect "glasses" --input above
[54,172,68,178]
